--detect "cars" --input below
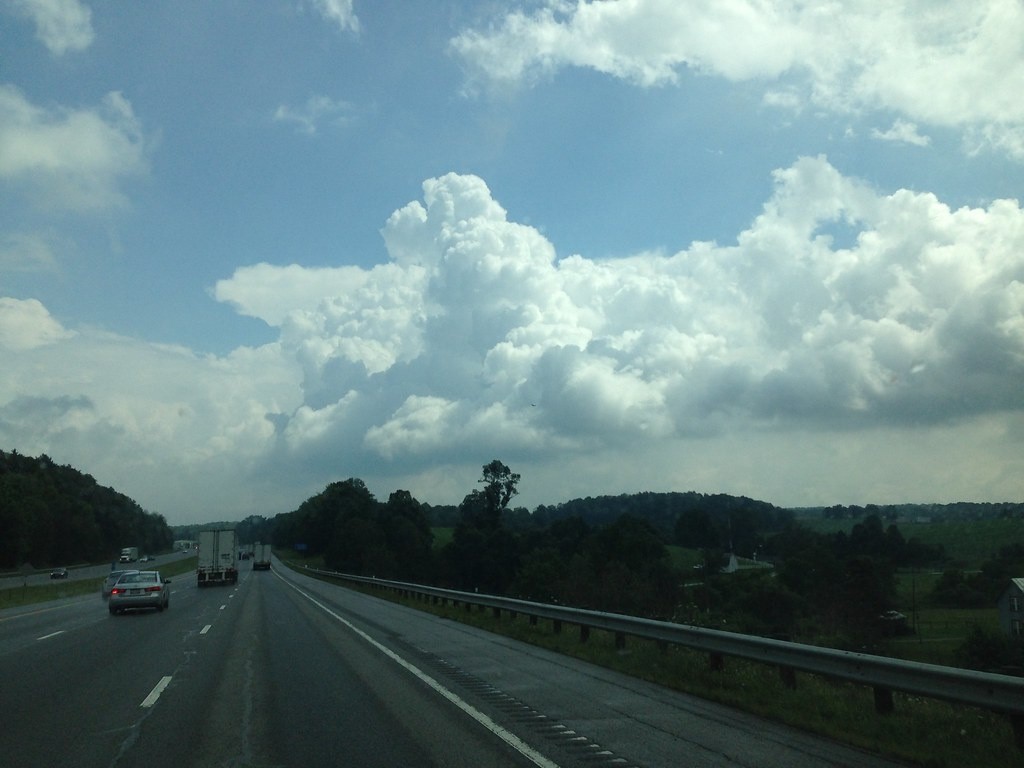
[239,549,250,560]
[110,571,172,614]
[50,568,68,579]
[102,571,138,598]
[140,555,150,562]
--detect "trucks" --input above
[253,544,272,570]
[196,529,239,587]
[120,548,138,563]
